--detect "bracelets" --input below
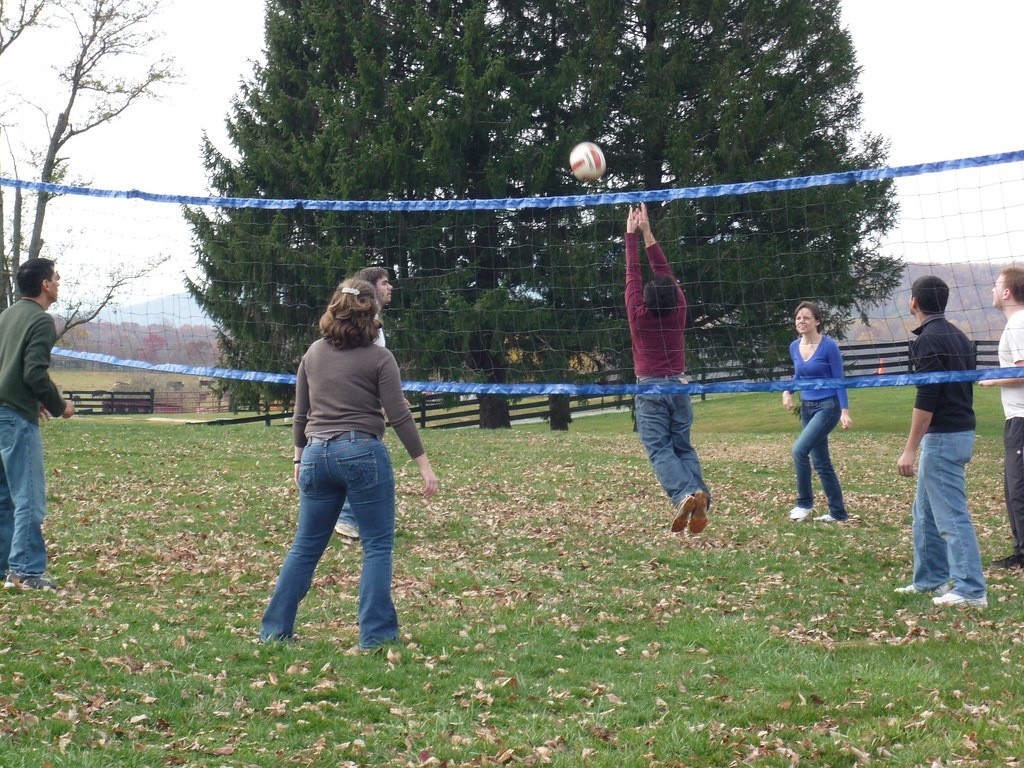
[291,459,302,464]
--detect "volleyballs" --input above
[569,142,606,181]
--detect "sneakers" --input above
[991,555,1024,570]
[931,591,988,608]
[4,573,56,594]
[894,582,950,597]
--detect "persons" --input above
[782,302,853,523]
[0,258,75,591]
[893,274,988,610]
[260,278,440,656]
[979,269,1024,570]
[335,268,395,539]
[625,202,712,533]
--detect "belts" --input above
[307,431,370,443]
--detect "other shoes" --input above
[690,491,709,533]
[790,506,811,520]
[814,514,835,522]
[670,495,697,532]
